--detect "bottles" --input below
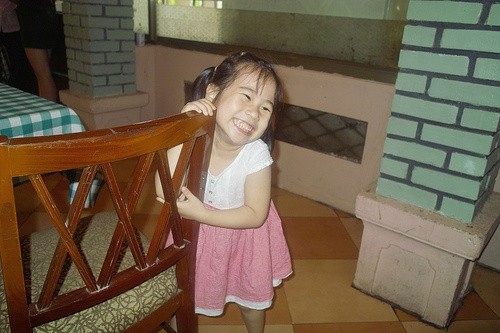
[136,23,145,46]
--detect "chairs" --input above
[0,106,218,333]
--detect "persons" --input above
[154,48,293,333]
[11,0,59,103]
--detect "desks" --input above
[0,83,101,210]
